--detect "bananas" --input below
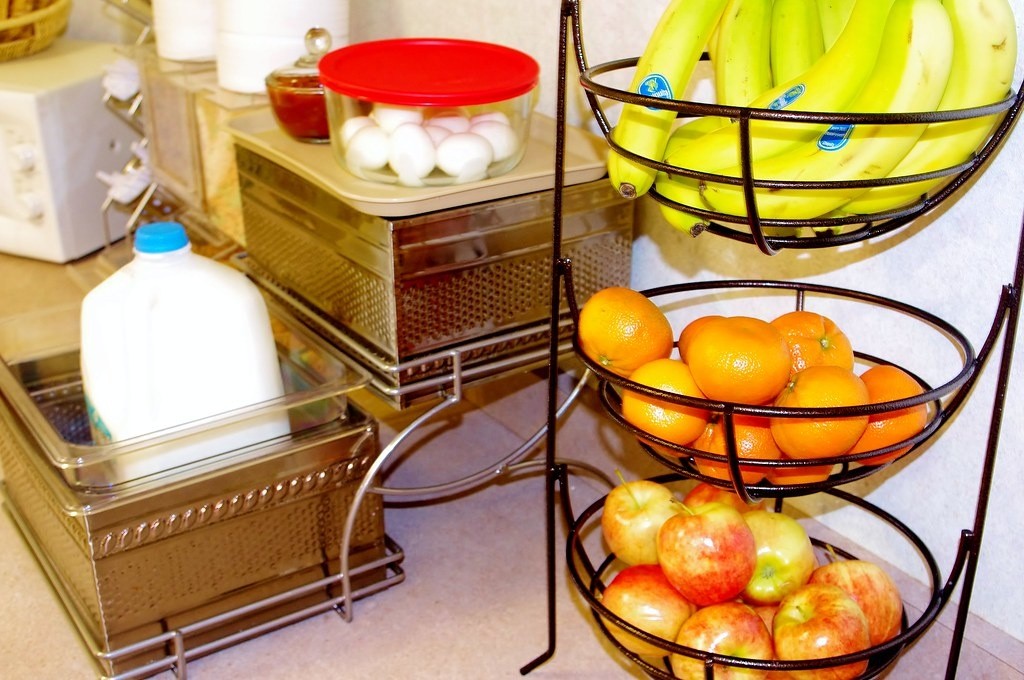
[604,0,1017,246]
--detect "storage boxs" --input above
[0,297,406,679]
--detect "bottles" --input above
[79,222,291,494]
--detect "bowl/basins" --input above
[317,38,540,188]
[265,29,373,145]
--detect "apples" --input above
[596,465,905,680]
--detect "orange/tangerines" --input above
[579,286,927,486]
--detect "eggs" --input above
[340,99,521,181]
